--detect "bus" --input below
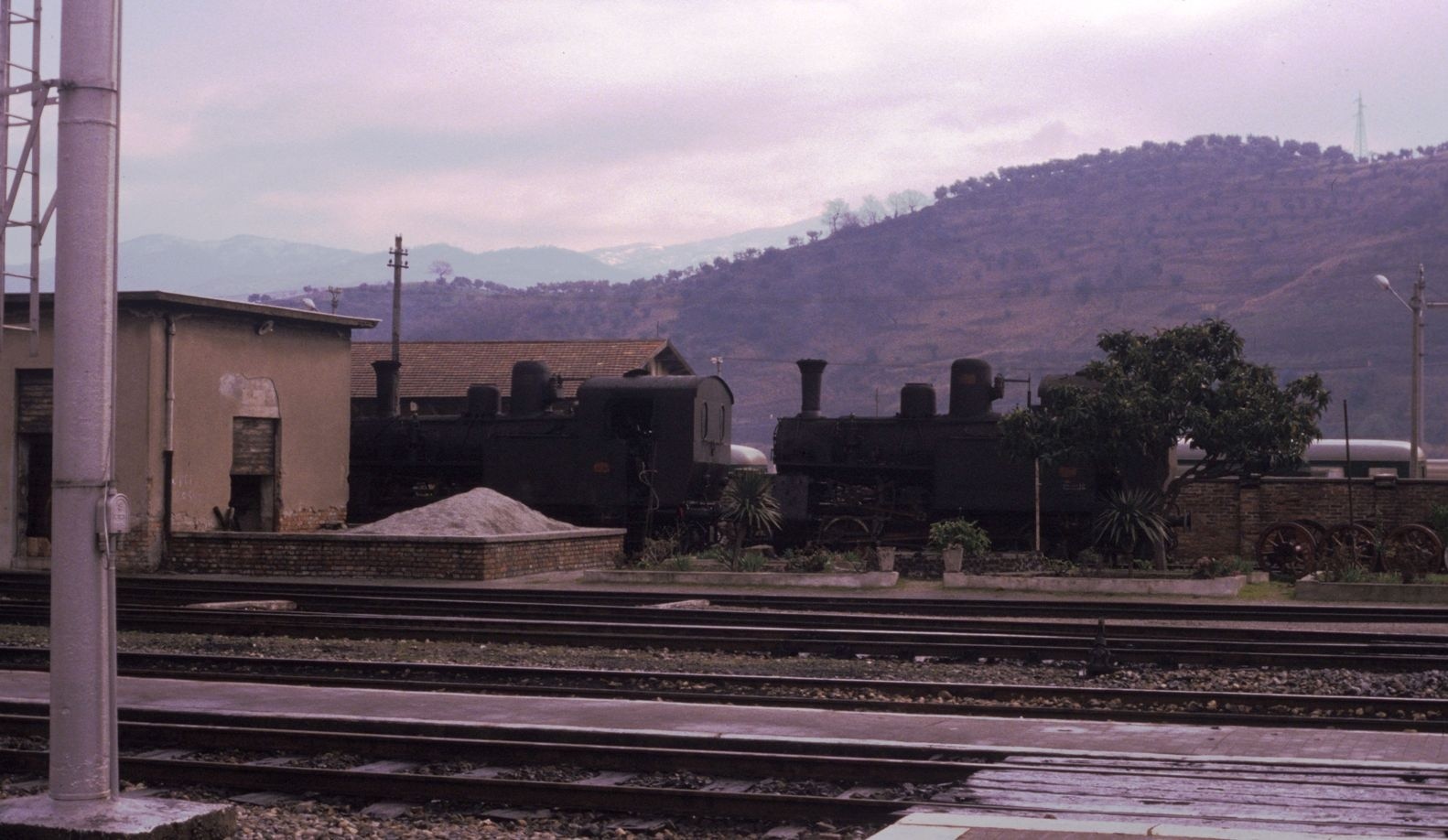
[1169,434,1428,482]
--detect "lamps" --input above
[258,318,275,335]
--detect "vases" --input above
[878,546,896,571]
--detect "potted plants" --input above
[927,514,991,573]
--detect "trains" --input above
[349,355,1030,551]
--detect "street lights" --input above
[1375,259,1448,483]
